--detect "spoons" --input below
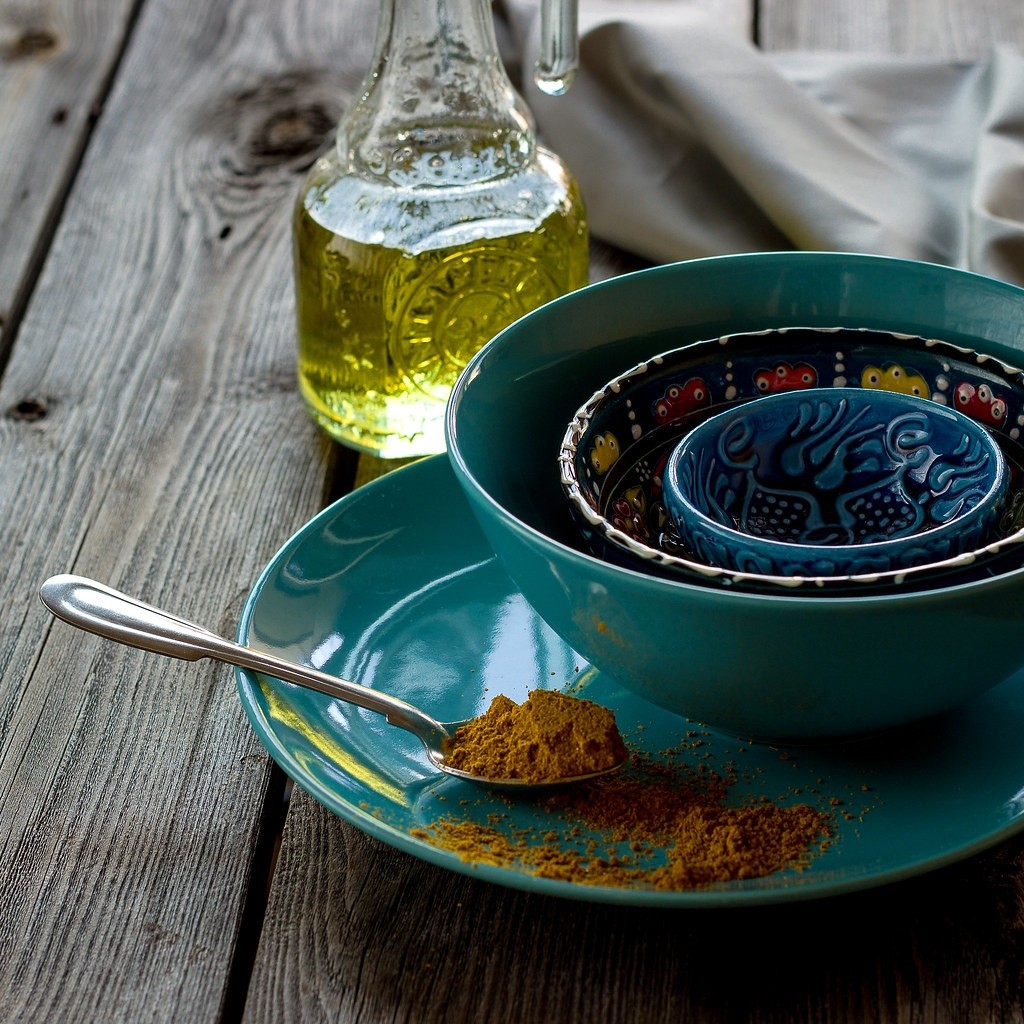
[39,573,627,789]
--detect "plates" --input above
[235,452,1023,908]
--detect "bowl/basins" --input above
[445,251,1024,744]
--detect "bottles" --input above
[292,0,588,461]
